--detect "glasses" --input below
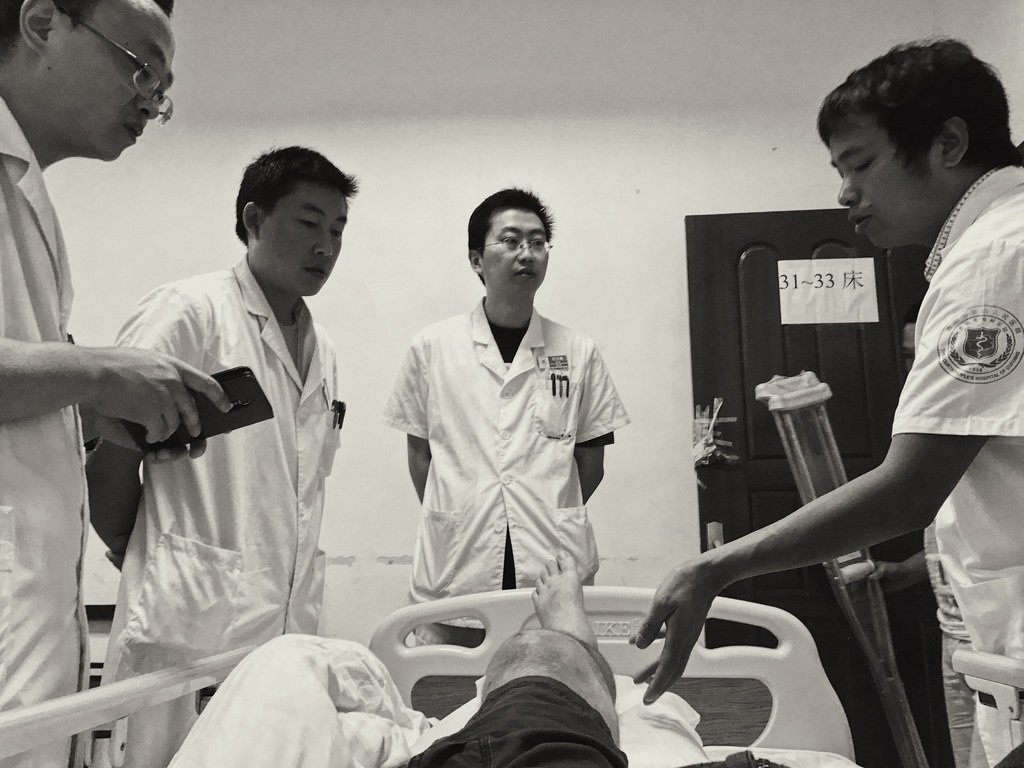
[56,5,174,127]
[478,237,553,254]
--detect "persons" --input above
[396,551,788,768]
[0,0,232,768]
[630,39,1024,768]
[381,189,631,648]
[85,146,359,768]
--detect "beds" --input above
[1,585,868,768]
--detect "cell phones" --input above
[121,367,274,454]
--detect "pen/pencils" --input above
[330,399,346,430]
[67,333,76,345]
[557,375,563,397]
[563,376,570,398]
[551,373,556,396]
